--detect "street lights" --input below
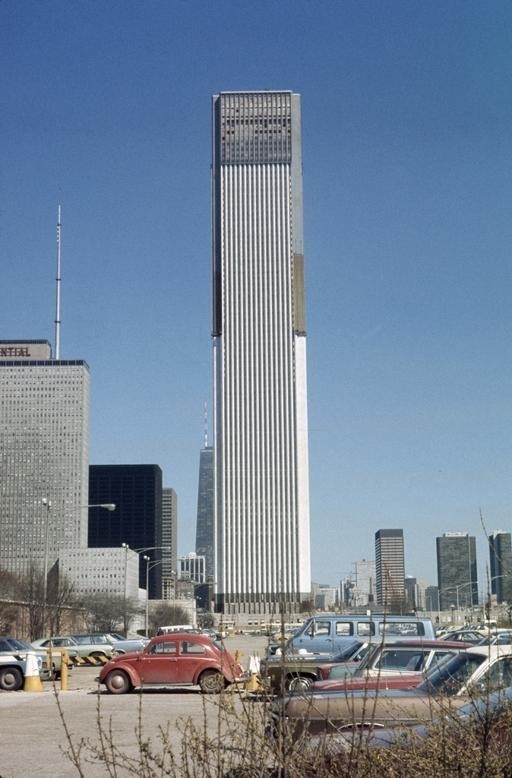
[40,494,118,626]
[117,537,205,637]
[436,573,509,610]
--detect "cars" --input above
[0,623,248,694]
[266,631,512,777]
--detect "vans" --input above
[257,614,437,696]
